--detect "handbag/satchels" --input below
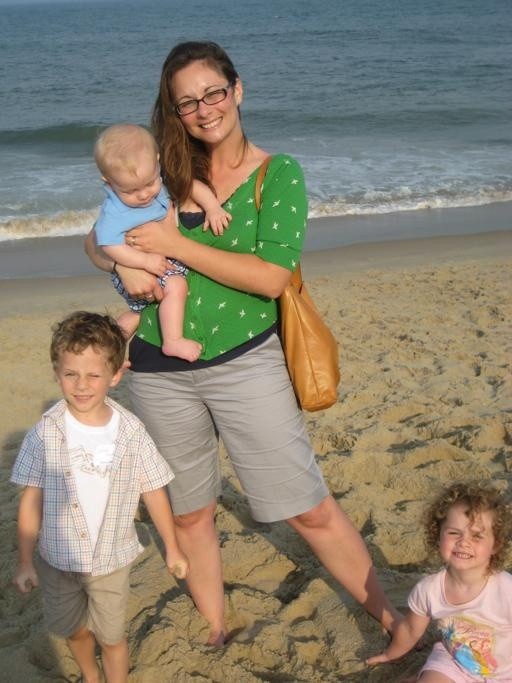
[278,262,341,413]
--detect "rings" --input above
[147,294,153,299]
[132,236,136,245]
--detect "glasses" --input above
[176,81,232,117]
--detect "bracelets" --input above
[112,262,118,272]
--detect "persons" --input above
[94,122,233,388]
[366,481,512,683]
[84,40,429,652]
[9,310,190,683]
